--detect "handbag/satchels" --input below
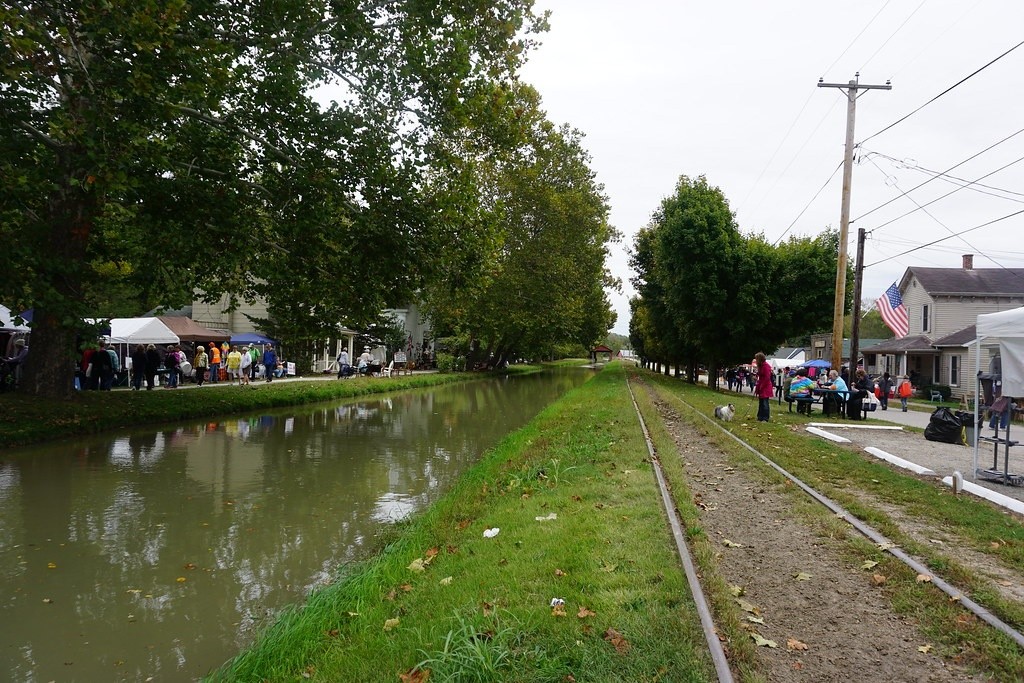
[879,392,885,397]
[191,368,197,377]
[337,353,341,362]
[862,391,876,411]
[255,365,259,373]
[86,362,92,378]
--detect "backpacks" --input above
[164,353,176,368]
[899,380,912,396]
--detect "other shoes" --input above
[133,386,136,390]
[883,405,887,410]
[903,406,907,413]
[164,384,177,389]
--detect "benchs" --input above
[795,398,816,416]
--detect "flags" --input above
[876,282,909,339]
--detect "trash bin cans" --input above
[954,410,986,447]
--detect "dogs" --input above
[714,403,736,422]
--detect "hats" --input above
[209,342,214,347]
[16,339,26,347]
[249,343,253,348]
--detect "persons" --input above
[723,367,757,393]
[756,353,774,423]
[3,339,30,363]
[773,367,874,421]
[989,378,1008,431]
[878,372,893,410]
[910,370,918,386]
[82,339,288,391]
[414,345,432,370]
[898,375,911,412]
[337,348,379,372]
[642,356,647,369]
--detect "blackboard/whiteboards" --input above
[394,352,407,362]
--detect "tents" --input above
[973,307,1024,485]
[100,317,180,387]
[230,332,282,379]
[0,303,33,332]
[159,316,231,356]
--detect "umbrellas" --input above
[797,359,831,367]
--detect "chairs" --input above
[338,359,416,379]
[930,389,943,403]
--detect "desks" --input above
[813,388,852,418]
